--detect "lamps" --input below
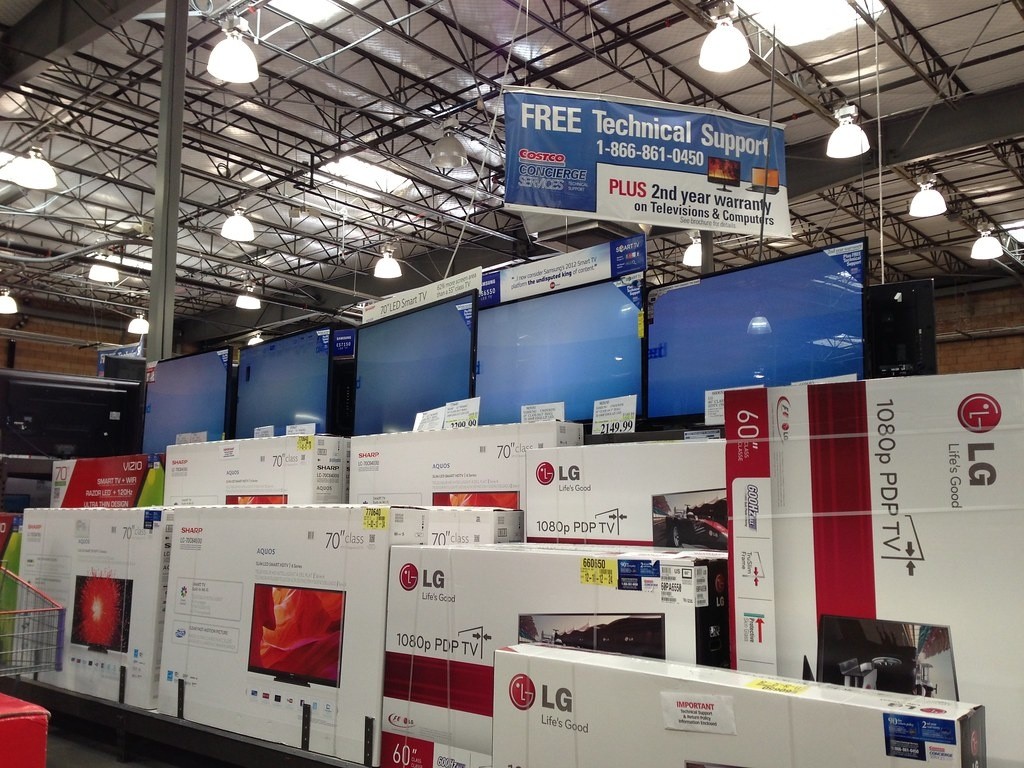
[0,286,18,315]
[206,14,260,84]
[698,4,750,74]
[827,105,871,158]
[429,118,469,167]
[220,203,255,242]
[908,173,947,217]
[682,231,702,267]
[11,141,57,189]
[89,249,119,282]
[970,221,1003,260]
[127,311,149,335]
[235,280,261,310]
[374,243,402,278]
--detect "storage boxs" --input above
[0,368,1024,768]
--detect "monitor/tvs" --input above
[0,368,142,461]
[816,615,959,704]
[142,237,937,463]
[518,612,666,661]
[433,491,520,510]
[70,575,133,653]
[248,583,346,688]
[226,494,288,506]
[652,489,729,552]
